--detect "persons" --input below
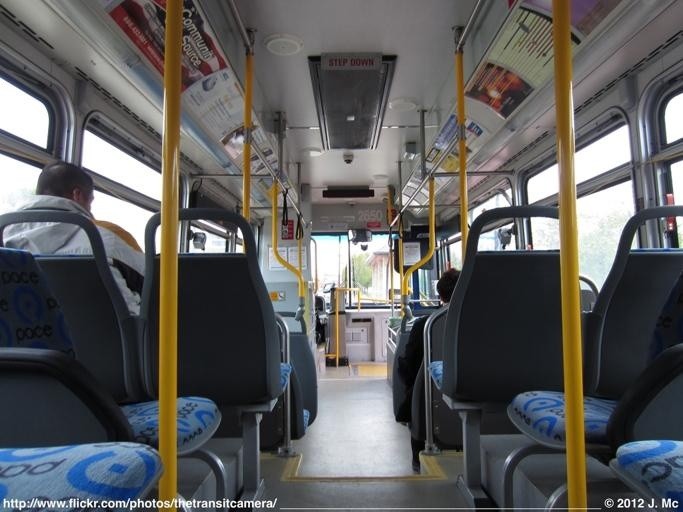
[395,269,461,423]
[3,164,145,319]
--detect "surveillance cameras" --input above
[343,154,353,164]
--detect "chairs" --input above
[0,207,317,511]
[393,203,683,512]
